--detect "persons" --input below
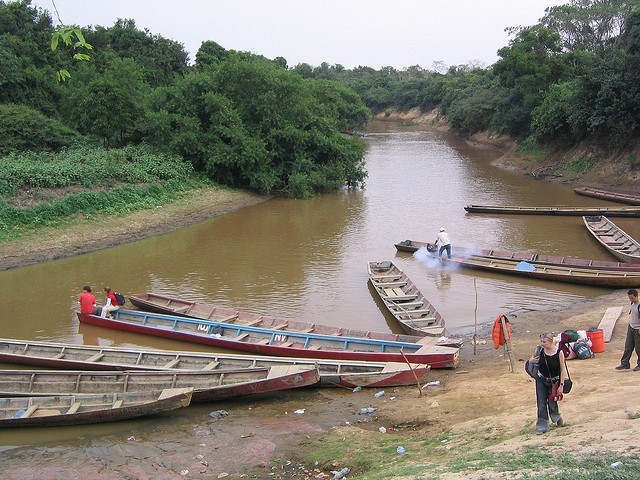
[434,227,452,259]
[78,286,101,315]
[101,286,121,319]
[615,289,640,371]
[528,330,567,435]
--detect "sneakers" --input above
[615,364,630,369]
[633,365,639,371]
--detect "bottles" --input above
[361,408,376,414]
[352,387,363,393]
[374,391,385,397]
[333,467,351,479]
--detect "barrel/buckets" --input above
[586,328,604,353]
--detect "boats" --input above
[394,240,640,272]
[573,186,640,206]
[128,291,464,347]
[0,388,194,429]
[425,244,640,288]
[581,215,640,264]
[1,336,431,387]
[463,203,640,216]
[366,260,447,336]
[76,305,460,369]
[0,362,320,400]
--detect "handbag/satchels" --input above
[543,380,563,401]
[561,330,579,342]
[573,339,595,359]
[558,377,572,393]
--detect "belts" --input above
[537,370,559,382]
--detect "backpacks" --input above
[525,346,543,378]
[114,292,125,306]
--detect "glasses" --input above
[539,334,550,339]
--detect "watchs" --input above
[560,382,564,386]
